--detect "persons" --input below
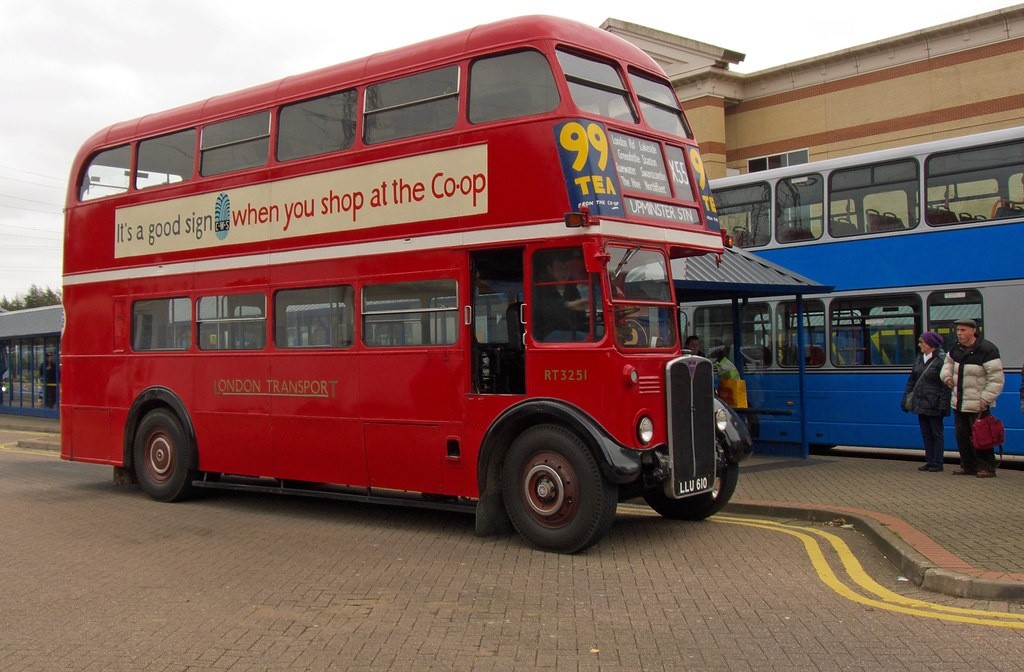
[940,318,1005,477]
[533,257,591,339]
[901,332,951,472]
[683,335,706,356]
[39,352,59,405]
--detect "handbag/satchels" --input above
[719,379,748,408]
[972,407,1005,450]
[714,356,740,380]
[904,392,914,410]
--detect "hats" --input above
[548,252,570,264]
[920,332,944,348]
[953,319,977,327]
[47,352,53,356]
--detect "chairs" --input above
[730,199,1024,247]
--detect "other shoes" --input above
[929,465,944,472]
[918,462,930,471]
[953,466,973,475]
[976,469,996,478]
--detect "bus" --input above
[59,15,753,556]
[709,126,1024,462]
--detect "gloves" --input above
[938,409,946,418]
[901,405,908,412]
